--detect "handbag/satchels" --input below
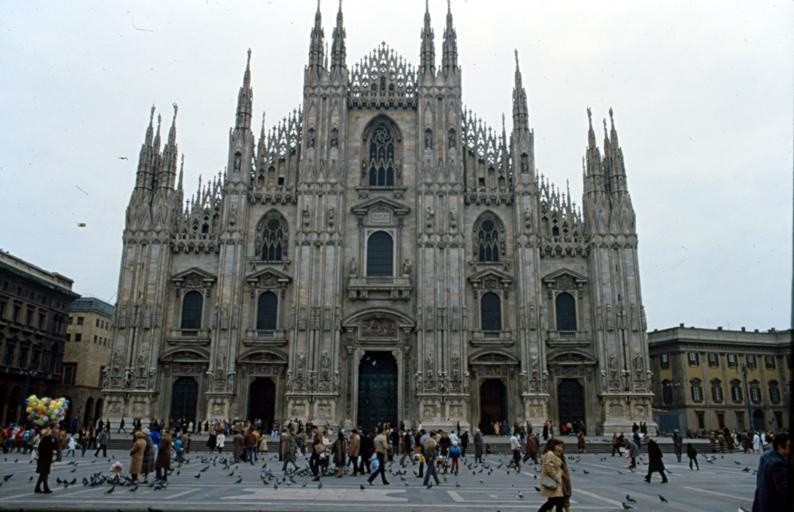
[542,476,557,490]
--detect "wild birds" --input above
[0,448,540,495]
[625,494,636,503]
[666,470,673,474]
[572,469,576,471]
[582,469,589,474]
[658,494,668,503]
[515,489,523,498]
[623,502,634,510]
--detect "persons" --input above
[0,416,793,512]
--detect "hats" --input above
[134,428,150,438]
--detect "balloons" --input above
[24,393,70,430]
